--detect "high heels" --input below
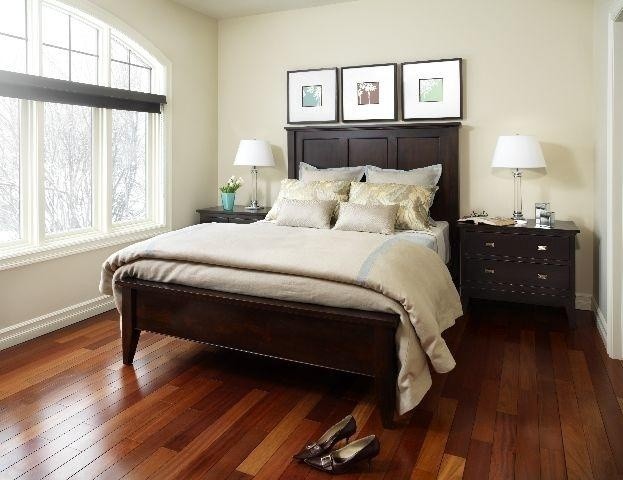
[292,414,356,459]
[304,435,380,474]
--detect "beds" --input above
[98,121,462,429]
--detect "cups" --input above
[539,212,556,228]
[534,202,551,224]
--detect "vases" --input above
[221,192,234,211]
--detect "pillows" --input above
[349,180,439,234]
[364,163,442,228]
[298,161,365,193]
[264,179,355,226]
[330,202,400,235]
[276,199,337,230]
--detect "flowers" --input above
[220,176,244,193]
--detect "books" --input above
[457,216,516,226]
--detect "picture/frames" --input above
[400,57,464,121]
[340,62,399,120]
[287,66,338,124]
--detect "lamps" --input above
[233,140,277,210]
[493,132,545,218]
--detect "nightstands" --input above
[450,215,582,329]
[196,203,272,224]
